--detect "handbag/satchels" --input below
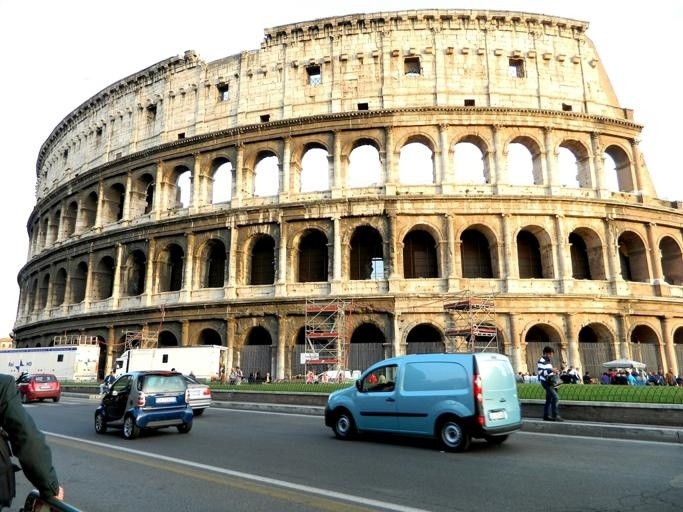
[545,376,559,387]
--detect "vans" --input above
[325,353,524,451]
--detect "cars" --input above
[162,374,213,418]
[98,374,120,393]
[93,371,194,442]
[14,372,63,404]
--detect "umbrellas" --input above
[602,357,645,368]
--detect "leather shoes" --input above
[544,416,564,421]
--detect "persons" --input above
[219,362,244,385]
[306,369,347,384]
[99,371,115,395]
[537,346,566,422]
[0,373,64,511]
[368,369,386,384]
[514,364,683,386]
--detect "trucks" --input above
[109,344,228,385]
